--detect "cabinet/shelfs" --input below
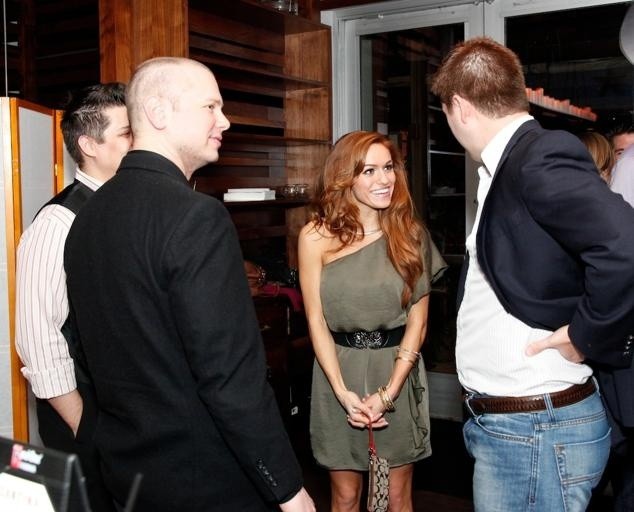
[110,0,335,370]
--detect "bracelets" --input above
[378,385,396,412]
[394,346,421,367]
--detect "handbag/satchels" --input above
[367,416,390,511]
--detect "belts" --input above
[461,376,596,417]
[329,325,405,349]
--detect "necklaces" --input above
[342,228,381,236]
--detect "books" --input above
[221,190,276,202]
[227,187,270,193]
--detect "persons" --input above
[15,85,133,510]
[429,37,633,511]
[295,131,447,512]
[575,124,634,205]
[64,56,320,511]
